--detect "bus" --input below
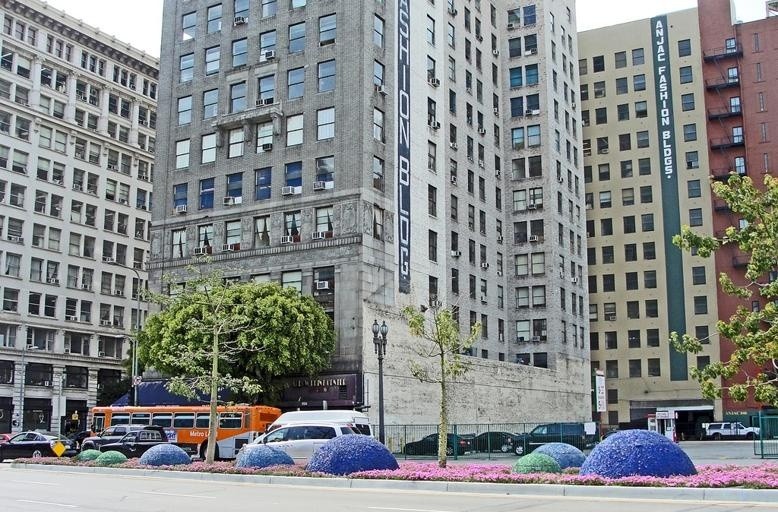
[90,404,282,461]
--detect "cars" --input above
[511,422,586,456]
[706,421,760,440]
[238,425,362,466]
[471,432,520,453]
[404,433,469,455]
[0,431,77,462]
[81,425,168,452]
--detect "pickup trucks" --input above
[99,430,198,459]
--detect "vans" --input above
[254,410,374,443]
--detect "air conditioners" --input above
[482,263,489,269]
[451,176,457,183]
[313,182,325,190]
[453,251,461,257]
[432,121,441,128]
[451,9,457,17]
[282,186,295,196]
[176,205,186,212]
[234,17,245,25]
[432,301,442,307]
[265,51,275,58]
[194,247,206,254]
[222,244,234,251]
[453,142,457,149]
[495,171,500,177]
[255,97,274,106]
[609,316,617,320]
[478,160,484,168]
[571,278,579,283]
[312,232,325,239]
[479,128,486,135]
[481,296,487,303]
[262,144,272,150]
[0,183,122,387]
[492,50,500,55]
[506,9,593,182]
[527,204,537,209]
[281,236,293,244]
[377,85,388,95]
[533,336,541,342]
[432,79,440,87]
[223,197,235,206]
[529,236,539,242]
[494,108,499,115]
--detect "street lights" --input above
[372,319,388,444]
[114,334,135,386]
[106,261,141,405]
